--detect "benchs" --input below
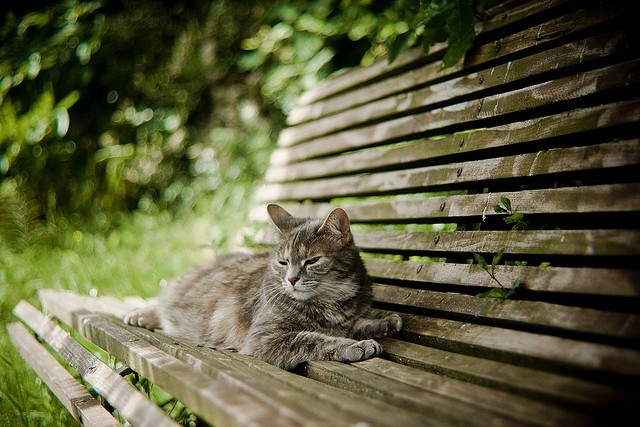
[6,1,638,426]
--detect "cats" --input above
[117,201,407,372]
